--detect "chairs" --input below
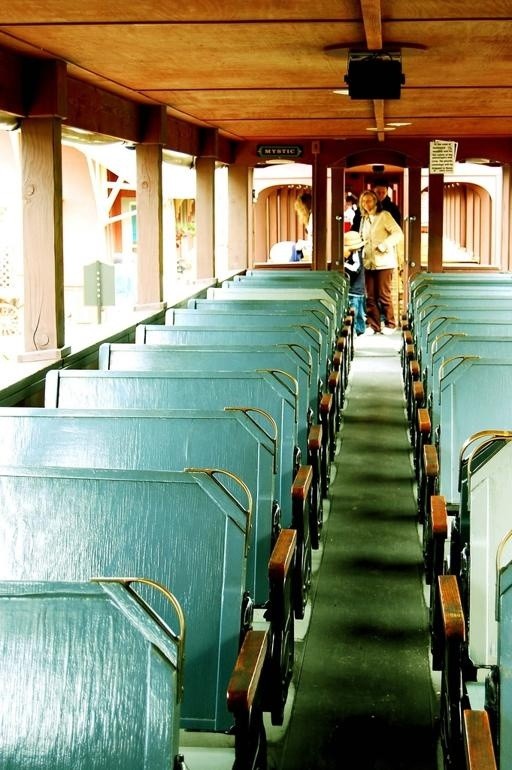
[381,327,398,334]
[365,327,380,335]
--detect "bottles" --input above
[343,231,367,250]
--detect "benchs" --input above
[398,266,512,770]
[1,265,355,770]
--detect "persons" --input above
[294,193,313,261]
[341,190,368,341]
[350,176,401,236]
[356,189,405,337]
